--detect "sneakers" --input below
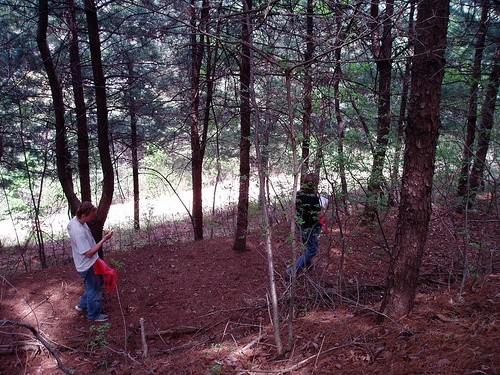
[75,305,88,312]
[87,315,110,322]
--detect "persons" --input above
[66,202,116,322]
[285,172,327,278]
